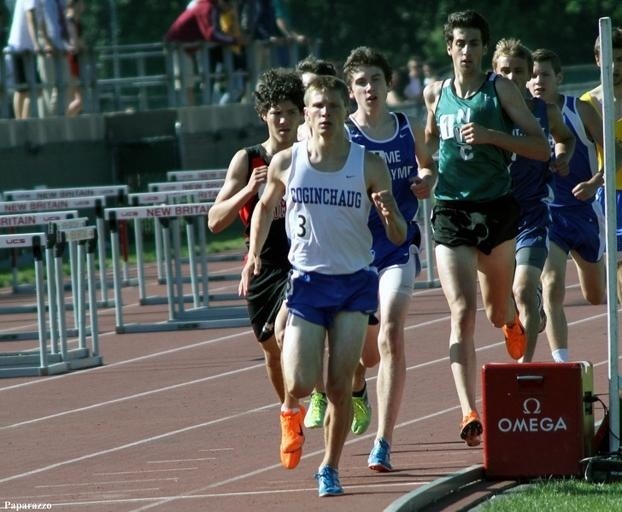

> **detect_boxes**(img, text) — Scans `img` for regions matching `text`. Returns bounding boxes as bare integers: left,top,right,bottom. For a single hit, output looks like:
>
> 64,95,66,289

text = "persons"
164,0,247,105
208,0,242,103
272,0,306,68
525,47,607,362
296,52,379,435
491,37,576,363
36,1,78,118
66,1,84,117
385,68,410,106
579,27,622,305
403,55,424,99
423,8,551,447
342,46,438,473
422,62,438,87
237,74,408,498
8,1,53,119
207,67,304,470
249,0,286,77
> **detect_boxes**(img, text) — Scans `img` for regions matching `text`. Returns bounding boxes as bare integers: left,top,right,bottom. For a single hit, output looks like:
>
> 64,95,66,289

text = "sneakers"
279,405,307,452
459,411,483,447
304,393,328,428
537,286,547,333
314,464,344,498
368,437,392,472
503,312,527,360
351,390,371,435
280,447,302,469
